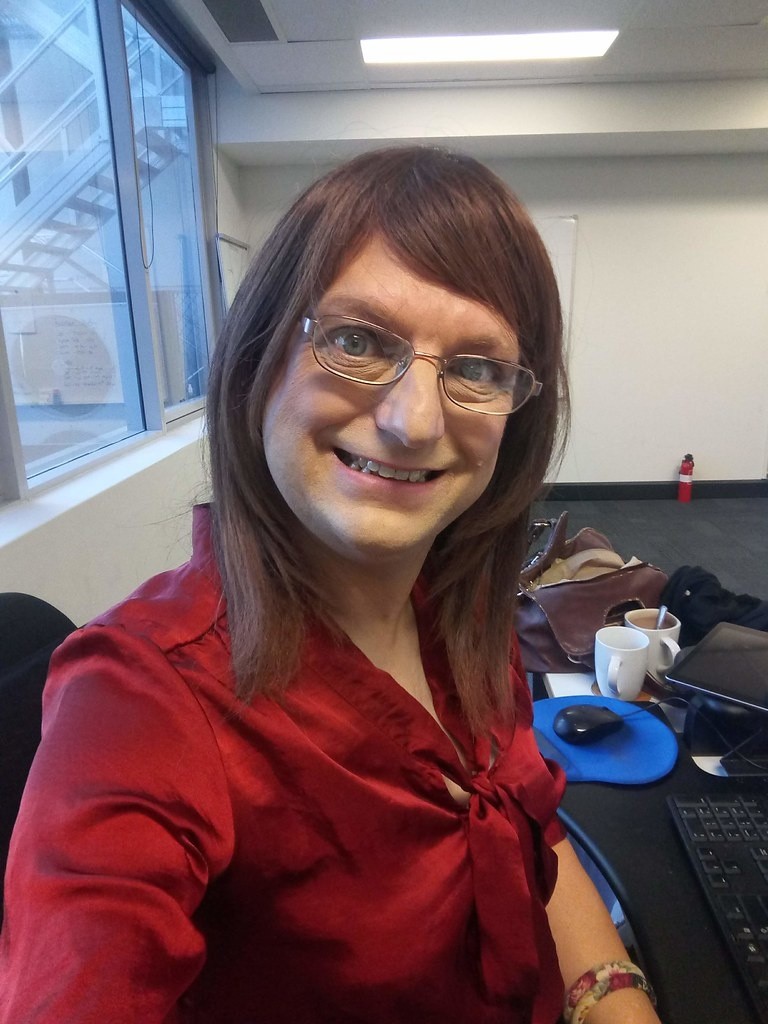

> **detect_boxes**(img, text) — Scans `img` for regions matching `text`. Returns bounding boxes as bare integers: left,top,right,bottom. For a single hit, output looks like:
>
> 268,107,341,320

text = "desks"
528,672,767,1024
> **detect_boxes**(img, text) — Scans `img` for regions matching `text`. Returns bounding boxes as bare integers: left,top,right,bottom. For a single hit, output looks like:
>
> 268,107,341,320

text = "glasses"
299,314,544,417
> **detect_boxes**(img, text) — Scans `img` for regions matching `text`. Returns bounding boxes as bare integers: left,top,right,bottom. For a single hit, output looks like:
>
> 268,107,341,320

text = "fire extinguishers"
678,453,695,503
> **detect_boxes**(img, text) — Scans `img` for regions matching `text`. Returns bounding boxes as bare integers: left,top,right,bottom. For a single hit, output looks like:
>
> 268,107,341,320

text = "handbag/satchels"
514,509,671,673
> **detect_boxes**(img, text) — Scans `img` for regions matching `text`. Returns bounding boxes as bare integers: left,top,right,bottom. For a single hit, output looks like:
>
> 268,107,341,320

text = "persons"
0,147,661,1024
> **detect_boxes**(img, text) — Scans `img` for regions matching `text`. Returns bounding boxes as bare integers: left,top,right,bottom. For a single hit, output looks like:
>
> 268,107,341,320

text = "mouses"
553,705,624,743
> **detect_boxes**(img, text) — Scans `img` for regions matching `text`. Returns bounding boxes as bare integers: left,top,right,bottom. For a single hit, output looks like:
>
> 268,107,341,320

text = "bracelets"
570,972,658,1024
560,960,646,1024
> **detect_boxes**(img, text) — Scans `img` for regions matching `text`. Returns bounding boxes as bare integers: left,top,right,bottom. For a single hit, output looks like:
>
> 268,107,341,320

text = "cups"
624,608,682,683
595,626,650,702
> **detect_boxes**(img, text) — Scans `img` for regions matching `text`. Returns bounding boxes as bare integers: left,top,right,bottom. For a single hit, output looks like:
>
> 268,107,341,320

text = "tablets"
664,622,768,716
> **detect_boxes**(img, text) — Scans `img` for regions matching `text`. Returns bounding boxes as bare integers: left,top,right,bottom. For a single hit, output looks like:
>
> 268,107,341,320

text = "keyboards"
666,790,767,1024
